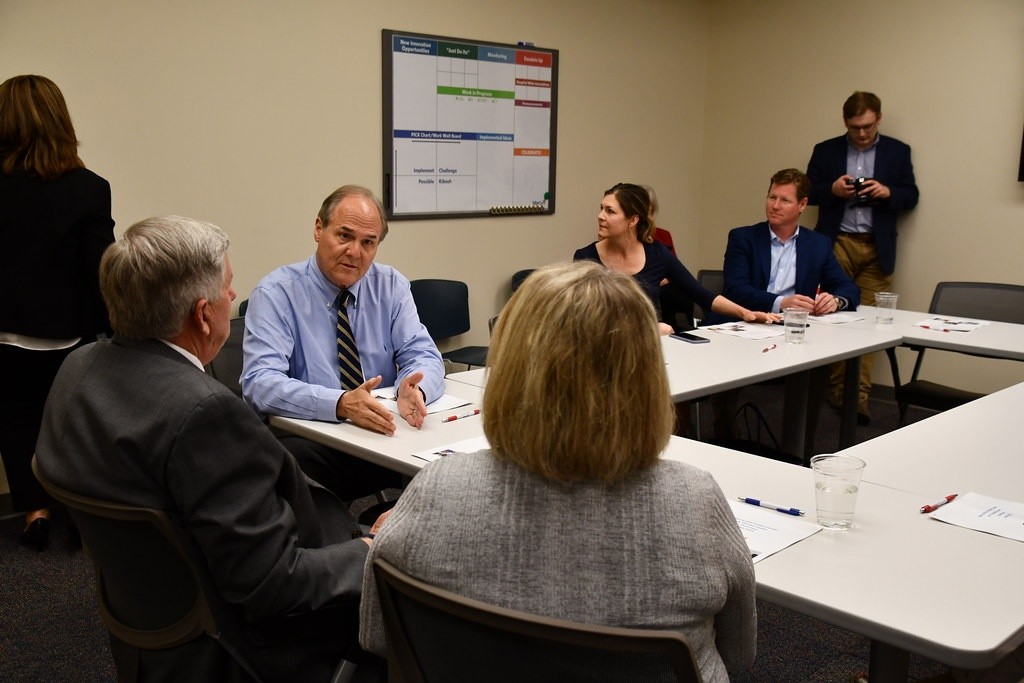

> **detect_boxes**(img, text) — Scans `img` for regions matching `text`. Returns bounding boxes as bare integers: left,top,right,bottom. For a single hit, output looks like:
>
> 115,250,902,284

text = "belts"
839,231,872,241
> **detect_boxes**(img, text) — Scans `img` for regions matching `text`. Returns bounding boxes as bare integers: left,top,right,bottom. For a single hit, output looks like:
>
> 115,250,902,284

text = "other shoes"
831,398,873,425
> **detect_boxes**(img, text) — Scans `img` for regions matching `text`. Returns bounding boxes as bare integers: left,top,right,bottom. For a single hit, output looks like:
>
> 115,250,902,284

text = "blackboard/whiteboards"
382,28,560,220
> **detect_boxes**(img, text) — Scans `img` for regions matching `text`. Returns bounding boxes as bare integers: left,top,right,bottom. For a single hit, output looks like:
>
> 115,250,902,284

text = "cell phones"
668,333,710,343
772,320,810,328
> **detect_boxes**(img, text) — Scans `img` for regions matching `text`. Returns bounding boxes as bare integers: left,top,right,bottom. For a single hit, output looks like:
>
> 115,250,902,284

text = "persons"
807,92,920,427
1,68,114,553
710,166,860,465
572,185,786,330
242,184,450,507
358,255,760,683
38,215,364,683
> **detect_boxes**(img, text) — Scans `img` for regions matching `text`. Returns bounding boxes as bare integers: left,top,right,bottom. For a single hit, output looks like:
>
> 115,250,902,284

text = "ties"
335,290,364,391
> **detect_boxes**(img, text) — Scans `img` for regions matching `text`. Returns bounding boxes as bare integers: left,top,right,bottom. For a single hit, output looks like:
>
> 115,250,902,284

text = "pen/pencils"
738,496,805,517
815,284,821,300
443,409,482,423
921,493,958,514
919,325,949,332
763,344,777,353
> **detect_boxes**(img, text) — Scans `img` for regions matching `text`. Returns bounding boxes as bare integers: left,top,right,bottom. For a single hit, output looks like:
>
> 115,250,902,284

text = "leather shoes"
23,508,51,545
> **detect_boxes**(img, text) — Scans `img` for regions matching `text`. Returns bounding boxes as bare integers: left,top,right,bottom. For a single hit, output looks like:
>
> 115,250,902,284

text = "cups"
810,454,866,529
782,307,810,344
875,292,900,324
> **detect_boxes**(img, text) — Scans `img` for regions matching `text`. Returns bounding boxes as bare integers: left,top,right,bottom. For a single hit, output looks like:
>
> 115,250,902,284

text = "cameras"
846,177,876,207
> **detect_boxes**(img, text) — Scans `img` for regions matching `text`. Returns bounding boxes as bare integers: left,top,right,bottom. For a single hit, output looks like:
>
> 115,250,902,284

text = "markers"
518,41,535,45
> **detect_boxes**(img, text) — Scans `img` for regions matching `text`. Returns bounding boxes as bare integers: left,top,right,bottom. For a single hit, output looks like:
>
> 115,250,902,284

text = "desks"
813,381,1024,502
446,320,908,465
269,379,1024,683
810,303,1024,362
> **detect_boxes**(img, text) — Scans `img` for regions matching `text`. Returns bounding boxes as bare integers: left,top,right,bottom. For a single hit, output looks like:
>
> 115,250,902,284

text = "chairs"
865,281,1024,423
369,554,703,683
202,317,244,399
30,455,364,683
402,267,537,373
